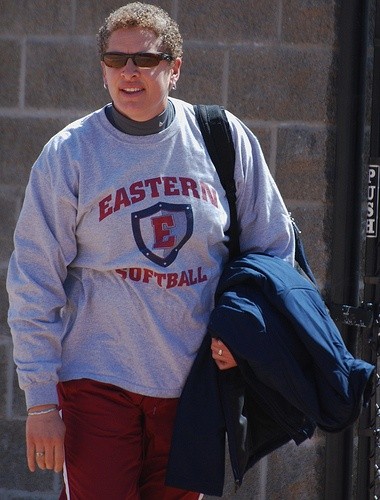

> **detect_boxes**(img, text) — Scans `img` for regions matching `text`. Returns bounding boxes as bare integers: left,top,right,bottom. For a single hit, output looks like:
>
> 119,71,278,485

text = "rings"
35,452,44,456
218,349,222,356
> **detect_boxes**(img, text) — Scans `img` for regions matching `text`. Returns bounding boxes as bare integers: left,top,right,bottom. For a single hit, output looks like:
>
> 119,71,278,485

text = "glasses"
102,52,173,68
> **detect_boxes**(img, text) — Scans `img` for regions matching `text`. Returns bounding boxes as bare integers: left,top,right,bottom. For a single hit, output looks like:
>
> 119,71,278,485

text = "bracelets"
27,407,58,416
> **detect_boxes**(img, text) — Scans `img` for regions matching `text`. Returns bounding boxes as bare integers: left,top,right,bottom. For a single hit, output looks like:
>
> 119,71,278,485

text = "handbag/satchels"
194,104,319,297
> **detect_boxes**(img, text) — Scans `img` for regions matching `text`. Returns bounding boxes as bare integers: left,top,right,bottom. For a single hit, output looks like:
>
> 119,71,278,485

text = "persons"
5,1,294,500
